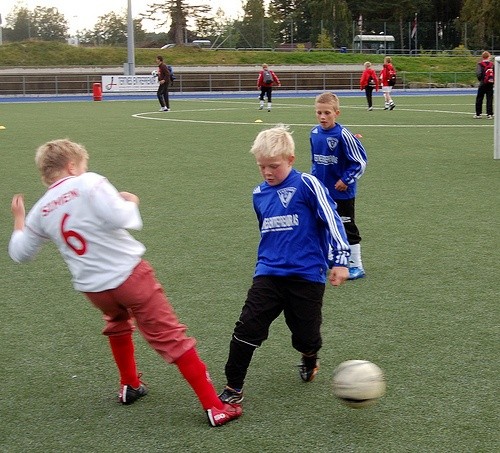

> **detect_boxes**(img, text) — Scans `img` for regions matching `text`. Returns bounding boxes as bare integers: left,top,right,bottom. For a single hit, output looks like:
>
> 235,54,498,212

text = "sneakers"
218,386,244,404
300,352,319,383
119,373,146,405
346,266,366,280
205,402,243,426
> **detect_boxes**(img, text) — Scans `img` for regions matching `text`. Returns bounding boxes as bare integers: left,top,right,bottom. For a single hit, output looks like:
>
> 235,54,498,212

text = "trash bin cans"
341,47,347,53
93,83,102,100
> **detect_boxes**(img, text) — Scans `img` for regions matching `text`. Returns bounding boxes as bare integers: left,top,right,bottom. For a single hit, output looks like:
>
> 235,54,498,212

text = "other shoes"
367,107,372,111
159,107,171,111
384,107,389,110
389,104,395,111
473,114,483,119
487,114,493,119
260,106,263,110
268,108,271,113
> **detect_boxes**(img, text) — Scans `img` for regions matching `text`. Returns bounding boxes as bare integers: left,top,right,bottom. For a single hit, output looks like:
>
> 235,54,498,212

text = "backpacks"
480,61,494,82
264,70,273,84
367,72,376,88
384,66,396,86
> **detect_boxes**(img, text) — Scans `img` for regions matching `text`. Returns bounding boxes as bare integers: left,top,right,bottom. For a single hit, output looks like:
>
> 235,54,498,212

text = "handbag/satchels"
166,65,175,80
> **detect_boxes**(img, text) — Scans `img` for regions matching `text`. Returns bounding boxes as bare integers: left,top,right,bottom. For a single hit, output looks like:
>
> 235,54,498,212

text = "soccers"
152,70,158,77
330,360,384,408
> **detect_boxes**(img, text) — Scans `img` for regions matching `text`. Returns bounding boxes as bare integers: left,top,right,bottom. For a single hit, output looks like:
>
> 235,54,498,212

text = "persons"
359,61,379,111
379,56,395,110
256,63,281,112
473,50,494,119
308,91,367,280
164,63,176,87
155,56,171,111
9,138,244,427
216,123,352,403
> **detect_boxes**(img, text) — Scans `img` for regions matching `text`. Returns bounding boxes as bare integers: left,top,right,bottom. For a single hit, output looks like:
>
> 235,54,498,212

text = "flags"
410,19,417,38
